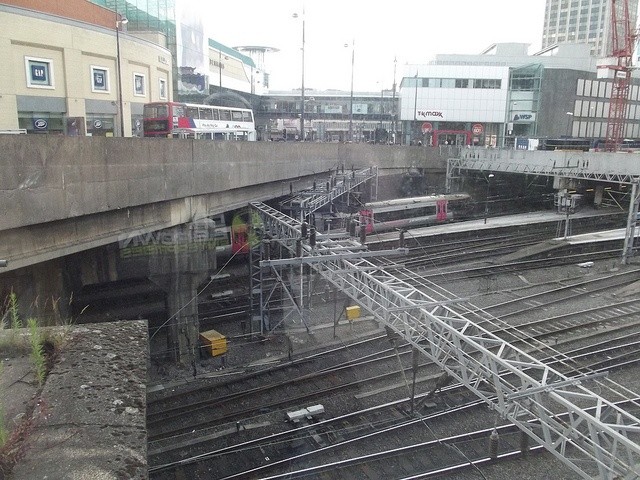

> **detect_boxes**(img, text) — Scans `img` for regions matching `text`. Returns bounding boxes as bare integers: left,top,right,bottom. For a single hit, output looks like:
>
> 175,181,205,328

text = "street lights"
344,38,355,136
116,18,130,138
292,12,305,142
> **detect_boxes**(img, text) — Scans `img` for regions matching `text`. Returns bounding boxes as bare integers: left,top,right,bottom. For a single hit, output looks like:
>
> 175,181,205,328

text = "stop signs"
472,124,483,136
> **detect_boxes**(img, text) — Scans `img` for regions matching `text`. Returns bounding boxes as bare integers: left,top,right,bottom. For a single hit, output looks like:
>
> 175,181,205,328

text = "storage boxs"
199,328,227,357
346,306,361,321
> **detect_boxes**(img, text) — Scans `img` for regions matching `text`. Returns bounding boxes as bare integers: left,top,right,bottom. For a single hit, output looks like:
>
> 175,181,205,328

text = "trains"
359,193,476,235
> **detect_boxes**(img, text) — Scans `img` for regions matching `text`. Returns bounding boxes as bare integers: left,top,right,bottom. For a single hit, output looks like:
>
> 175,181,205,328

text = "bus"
144,102,258,143
